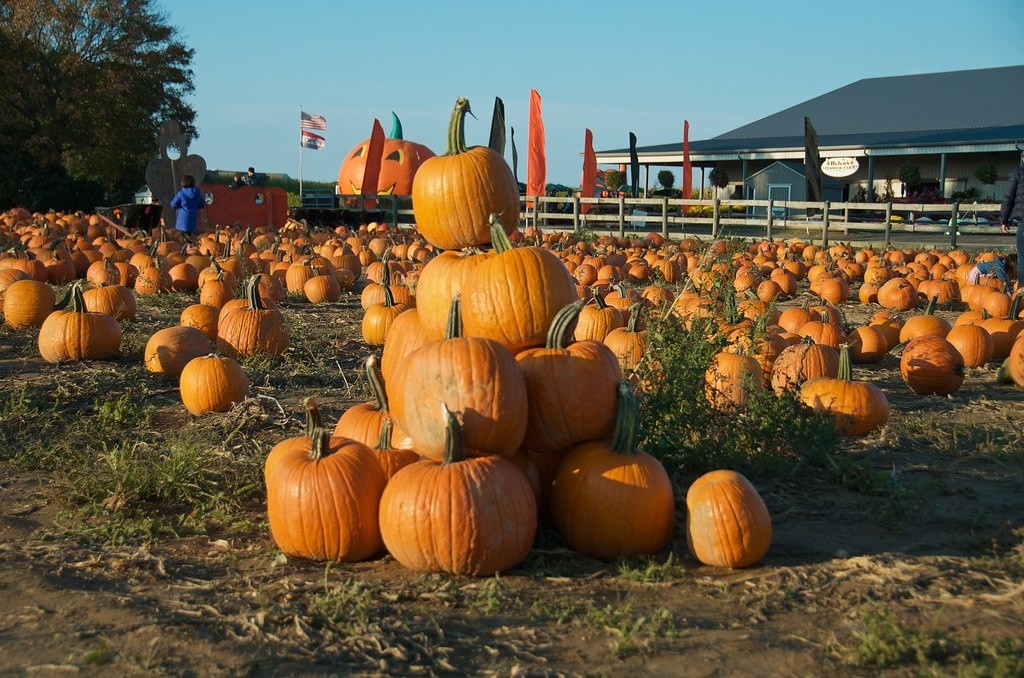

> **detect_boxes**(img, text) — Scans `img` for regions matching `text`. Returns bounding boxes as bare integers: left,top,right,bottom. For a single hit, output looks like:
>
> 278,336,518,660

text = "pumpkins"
0,97,1024,577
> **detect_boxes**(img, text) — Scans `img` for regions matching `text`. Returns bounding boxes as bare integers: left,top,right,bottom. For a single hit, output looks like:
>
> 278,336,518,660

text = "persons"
169,175,204,244
241,167,263,187
231,173,246,192
966,253,1018,284
999,151,1024,287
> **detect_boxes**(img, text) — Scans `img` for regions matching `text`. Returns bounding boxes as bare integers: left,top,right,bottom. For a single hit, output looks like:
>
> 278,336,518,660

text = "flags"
301,130,326,151
300,111,326,131
